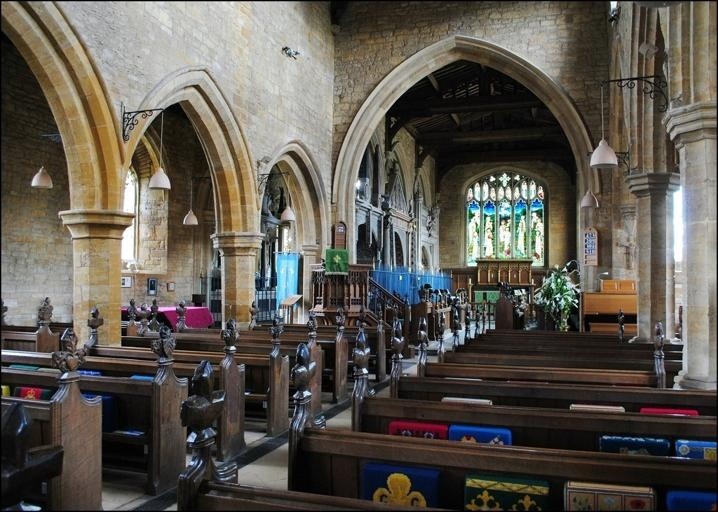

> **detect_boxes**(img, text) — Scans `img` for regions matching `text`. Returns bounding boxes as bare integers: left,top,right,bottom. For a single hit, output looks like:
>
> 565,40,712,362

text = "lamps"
120,103,172,194
578,150,631,209
27,128,70,194
182,171,215,229
586,71,669,170
255,168,299,226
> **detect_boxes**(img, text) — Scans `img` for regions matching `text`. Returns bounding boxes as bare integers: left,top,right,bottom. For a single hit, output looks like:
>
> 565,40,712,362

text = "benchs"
0,279,717,512
